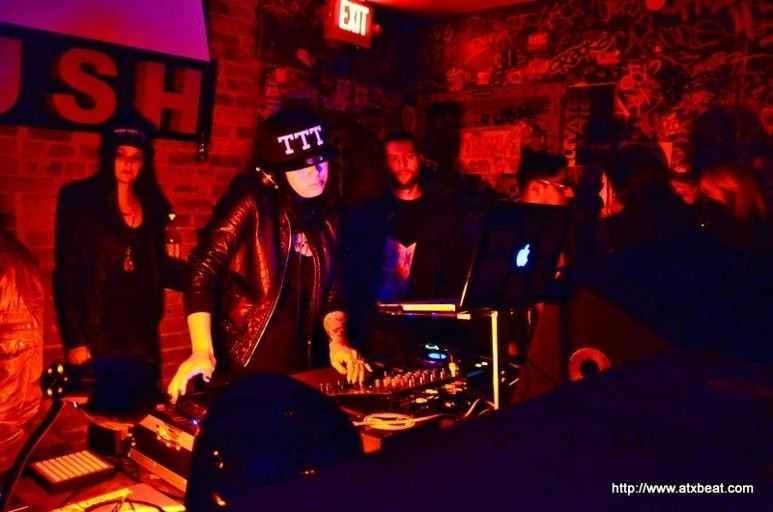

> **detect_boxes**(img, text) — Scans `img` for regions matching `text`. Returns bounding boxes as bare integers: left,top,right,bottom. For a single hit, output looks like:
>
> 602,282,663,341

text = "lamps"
0,355,162,512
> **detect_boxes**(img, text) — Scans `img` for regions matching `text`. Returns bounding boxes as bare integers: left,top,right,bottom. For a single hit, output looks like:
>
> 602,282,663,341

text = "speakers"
509,287,673,406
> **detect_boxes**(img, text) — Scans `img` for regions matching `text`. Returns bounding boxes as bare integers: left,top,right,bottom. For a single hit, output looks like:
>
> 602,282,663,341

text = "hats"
103,128,153,164
257,109,340,172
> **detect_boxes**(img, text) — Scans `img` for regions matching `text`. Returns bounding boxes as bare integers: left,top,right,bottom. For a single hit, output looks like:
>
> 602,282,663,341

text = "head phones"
255,164,282,192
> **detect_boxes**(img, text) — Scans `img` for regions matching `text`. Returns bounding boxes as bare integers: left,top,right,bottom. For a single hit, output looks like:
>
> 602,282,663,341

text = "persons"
53,121,189,452
340,129,458,355
163,103,372,403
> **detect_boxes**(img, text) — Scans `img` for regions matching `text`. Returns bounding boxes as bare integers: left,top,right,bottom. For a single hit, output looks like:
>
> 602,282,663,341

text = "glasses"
539,178,569,189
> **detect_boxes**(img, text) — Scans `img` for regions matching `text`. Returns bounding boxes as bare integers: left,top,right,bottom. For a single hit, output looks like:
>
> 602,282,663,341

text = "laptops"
378,202,573,312
